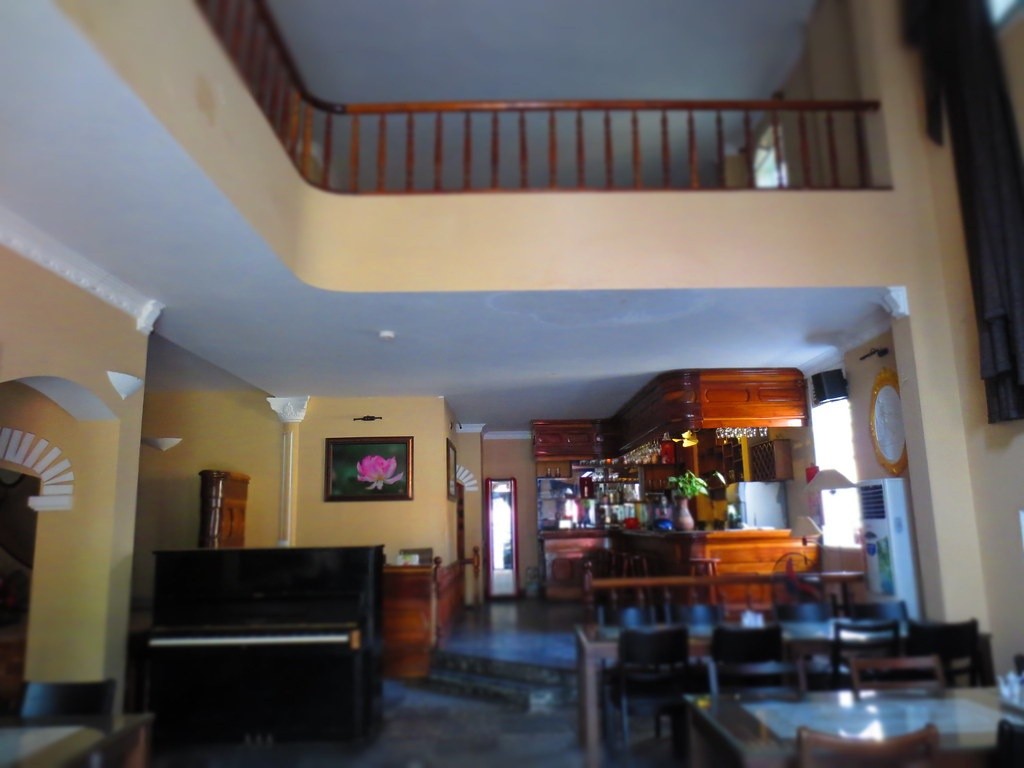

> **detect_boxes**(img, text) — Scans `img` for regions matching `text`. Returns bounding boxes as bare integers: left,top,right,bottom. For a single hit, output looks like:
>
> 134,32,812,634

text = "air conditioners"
857,476,923,624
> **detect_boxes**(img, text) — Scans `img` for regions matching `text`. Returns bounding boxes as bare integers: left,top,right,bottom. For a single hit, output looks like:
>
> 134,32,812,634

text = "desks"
684,684,1024,768
574,616,909,768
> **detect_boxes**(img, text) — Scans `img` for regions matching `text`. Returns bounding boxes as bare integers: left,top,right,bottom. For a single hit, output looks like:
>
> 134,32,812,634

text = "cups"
715,427,767,439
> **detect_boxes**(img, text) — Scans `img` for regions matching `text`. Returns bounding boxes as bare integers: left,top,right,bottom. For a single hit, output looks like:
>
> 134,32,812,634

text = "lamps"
811,368,851,405
801,467,860,496
579,431,676,465
680,430,698,447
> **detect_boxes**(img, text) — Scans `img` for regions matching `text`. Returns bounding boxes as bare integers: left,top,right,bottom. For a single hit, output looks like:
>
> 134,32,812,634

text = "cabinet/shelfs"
196,469,252,550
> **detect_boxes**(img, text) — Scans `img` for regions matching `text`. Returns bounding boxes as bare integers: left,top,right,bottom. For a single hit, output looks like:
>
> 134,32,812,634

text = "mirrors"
870,366,910,478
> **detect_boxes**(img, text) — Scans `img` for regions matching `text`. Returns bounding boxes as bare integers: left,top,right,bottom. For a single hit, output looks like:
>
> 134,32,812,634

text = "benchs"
591,570,867,618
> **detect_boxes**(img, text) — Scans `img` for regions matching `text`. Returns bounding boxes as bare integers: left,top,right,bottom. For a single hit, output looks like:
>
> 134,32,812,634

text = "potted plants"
669,471,709,530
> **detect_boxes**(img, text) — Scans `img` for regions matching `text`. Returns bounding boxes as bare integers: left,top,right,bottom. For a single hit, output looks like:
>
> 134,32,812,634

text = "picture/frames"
323,435,415,502
446,437,456,502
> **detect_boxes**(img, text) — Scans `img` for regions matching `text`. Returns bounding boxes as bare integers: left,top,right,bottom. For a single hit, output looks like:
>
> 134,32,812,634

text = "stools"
592,544,723,601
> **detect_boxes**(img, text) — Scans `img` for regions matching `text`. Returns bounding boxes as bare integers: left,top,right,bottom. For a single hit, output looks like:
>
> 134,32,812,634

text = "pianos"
144,543,389,752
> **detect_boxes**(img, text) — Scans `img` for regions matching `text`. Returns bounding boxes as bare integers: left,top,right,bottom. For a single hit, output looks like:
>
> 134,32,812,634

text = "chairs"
592,602,1024,768
